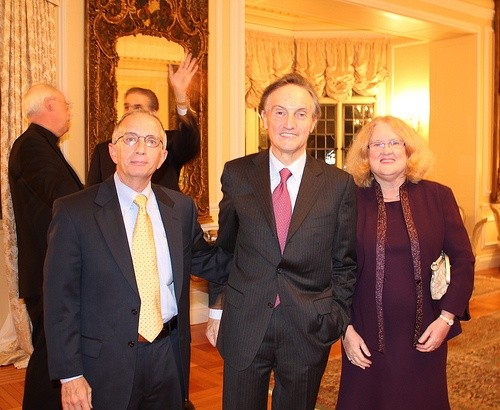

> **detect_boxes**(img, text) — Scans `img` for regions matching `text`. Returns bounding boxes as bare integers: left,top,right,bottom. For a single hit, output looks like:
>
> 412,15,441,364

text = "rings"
348,358,353,361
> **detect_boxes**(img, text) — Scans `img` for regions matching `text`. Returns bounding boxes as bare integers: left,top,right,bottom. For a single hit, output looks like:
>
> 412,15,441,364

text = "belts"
136,315,179,344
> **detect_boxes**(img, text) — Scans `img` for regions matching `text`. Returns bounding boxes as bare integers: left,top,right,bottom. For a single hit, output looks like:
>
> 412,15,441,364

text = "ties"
131,195,164,343
271,168,293,309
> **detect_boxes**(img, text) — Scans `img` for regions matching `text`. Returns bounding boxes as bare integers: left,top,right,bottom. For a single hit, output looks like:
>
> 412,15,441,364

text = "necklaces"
383,195,400,199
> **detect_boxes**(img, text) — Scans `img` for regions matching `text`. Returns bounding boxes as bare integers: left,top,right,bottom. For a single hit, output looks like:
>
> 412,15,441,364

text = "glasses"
114,132,163,150
47,97,70,111
368,137,405,152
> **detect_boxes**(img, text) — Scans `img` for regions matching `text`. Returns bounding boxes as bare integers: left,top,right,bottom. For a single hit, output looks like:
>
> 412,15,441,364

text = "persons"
206,73,357,410
7,83,85,410
84,52,201,191
336,115,475,410
44,110,218,410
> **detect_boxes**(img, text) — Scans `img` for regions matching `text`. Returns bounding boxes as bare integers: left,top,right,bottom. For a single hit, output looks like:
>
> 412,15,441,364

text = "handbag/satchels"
430,250,451,300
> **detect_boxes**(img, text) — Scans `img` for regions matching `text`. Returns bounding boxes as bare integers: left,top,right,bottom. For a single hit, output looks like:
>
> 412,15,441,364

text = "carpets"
269,310,500,410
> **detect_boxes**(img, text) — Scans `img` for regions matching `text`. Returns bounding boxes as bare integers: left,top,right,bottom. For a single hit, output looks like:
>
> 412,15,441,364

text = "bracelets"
174,99,188,105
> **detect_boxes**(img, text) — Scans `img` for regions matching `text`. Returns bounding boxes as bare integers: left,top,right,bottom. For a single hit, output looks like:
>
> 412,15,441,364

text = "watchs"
440,315,454,325
